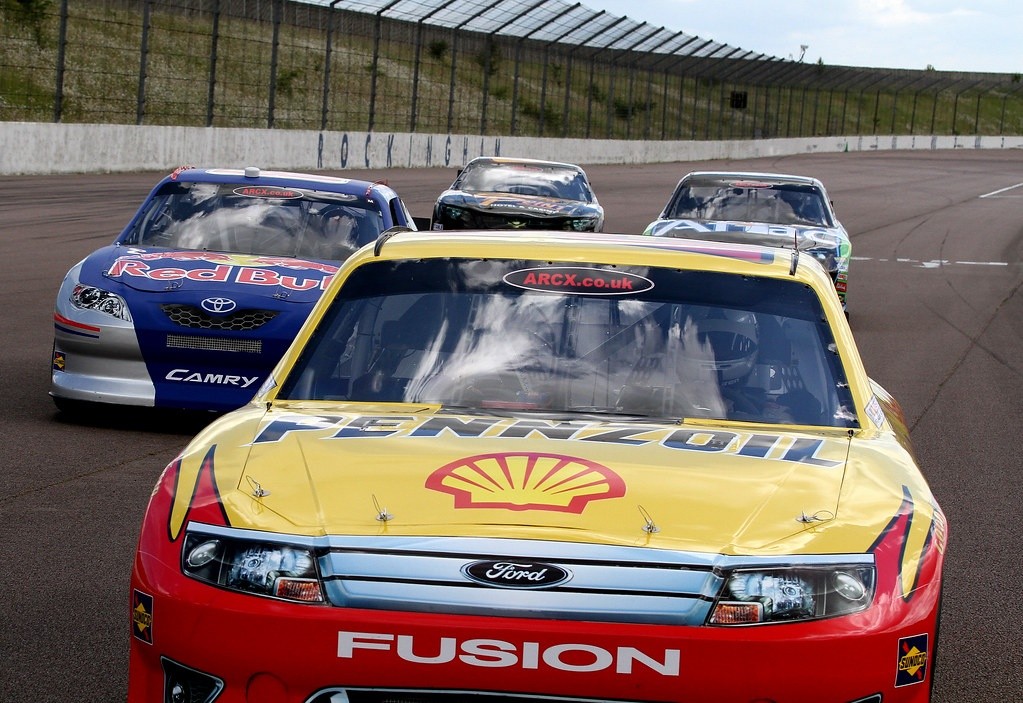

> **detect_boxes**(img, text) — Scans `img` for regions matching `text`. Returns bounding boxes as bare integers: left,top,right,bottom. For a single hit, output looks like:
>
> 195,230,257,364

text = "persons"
633,303,812,429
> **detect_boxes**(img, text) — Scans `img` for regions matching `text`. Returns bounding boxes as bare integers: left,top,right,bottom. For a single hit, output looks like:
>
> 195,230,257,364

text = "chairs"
358,291,493,403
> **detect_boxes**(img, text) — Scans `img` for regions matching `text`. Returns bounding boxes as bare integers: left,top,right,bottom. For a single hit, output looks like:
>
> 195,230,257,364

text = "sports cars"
127,224,953,703
643,171,853,321
429,157,606,237
49,165,420,418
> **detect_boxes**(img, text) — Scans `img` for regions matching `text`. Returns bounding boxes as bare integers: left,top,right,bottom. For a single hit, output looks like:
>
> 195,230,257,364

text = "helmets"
777,189,804,216
673,305,758,384
319,206,361,248
